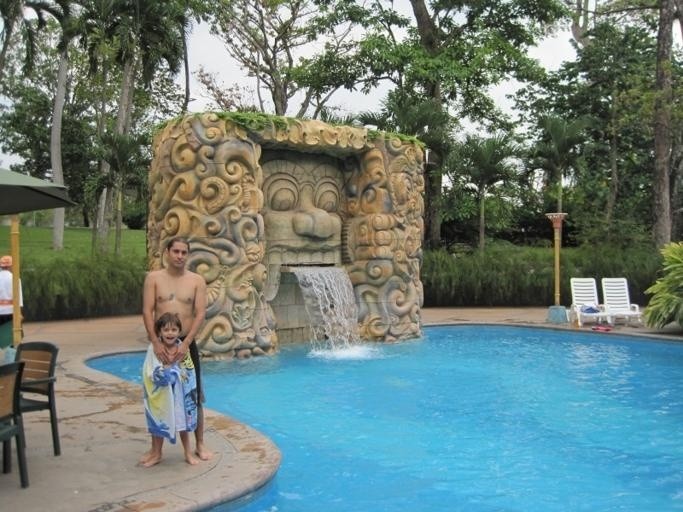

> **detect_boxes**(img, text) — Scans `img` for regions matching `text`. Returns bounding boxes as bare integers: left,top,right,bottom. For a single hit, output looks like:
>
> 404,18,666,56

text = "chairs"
14,341,61,456
566,277,610,327
601,277,644,328
0,360,30,488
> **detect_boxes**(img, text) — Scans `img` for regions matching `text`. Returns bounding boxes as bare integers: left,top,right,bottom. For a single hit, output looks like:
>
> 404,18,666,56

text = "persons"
140,314,200,468
1,255,25,351
138,240,213,463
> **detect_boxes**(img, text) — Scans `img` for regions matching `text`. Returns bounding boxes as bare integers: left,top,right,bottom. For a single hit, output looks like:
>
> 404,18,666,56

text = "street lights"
544,213,569,324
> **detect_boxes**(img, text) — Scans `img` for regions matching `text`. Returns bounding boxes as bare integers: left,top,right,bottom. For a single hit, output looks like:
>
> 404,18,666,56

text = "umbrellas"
0,166,79,216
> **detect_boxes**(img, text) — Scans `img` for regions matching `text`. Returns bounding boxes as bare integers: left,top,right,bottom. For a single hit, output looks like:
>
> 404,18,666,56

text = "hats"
0,254,13,268
